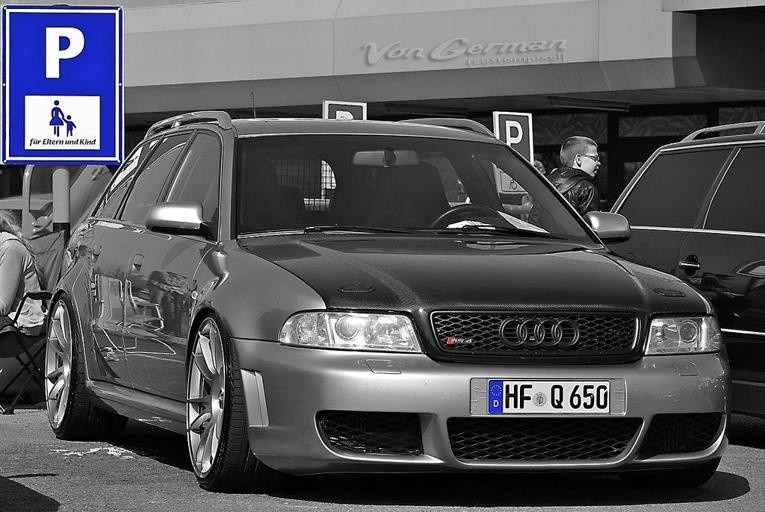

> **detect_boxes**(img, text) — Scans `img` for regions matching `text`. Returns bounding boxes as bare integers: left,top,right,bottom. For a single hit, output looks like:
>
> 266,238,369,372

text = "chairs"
1,291,57,415
238,161,451,231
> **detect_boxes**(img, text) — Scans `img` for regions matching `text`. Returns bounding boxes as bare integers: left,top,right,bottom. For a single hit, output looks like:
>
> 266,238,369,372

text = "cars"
40,91,734,496
595,118,765,426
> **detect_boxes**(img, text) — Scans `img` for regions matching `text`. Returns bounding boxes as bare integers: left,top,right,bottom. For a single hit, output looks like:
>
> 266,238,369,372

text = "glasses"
581,154,599,162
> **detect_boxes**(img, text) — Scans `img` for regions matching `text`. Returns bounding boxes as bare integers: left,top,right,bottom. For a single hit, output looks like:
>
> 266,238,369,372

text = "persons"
0,211,45,357
527,133,603,233
